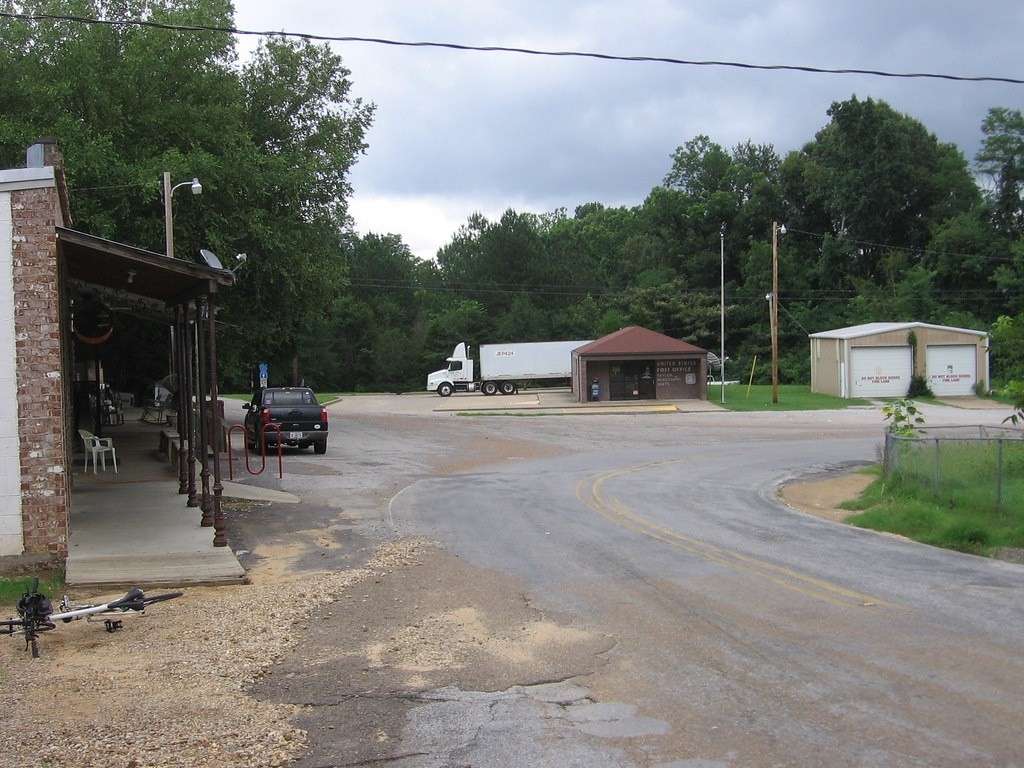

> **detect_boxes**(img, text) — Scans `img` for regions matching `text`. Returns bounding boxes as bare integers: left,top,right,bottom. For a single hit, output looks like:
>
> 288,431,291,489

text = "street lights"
772,222,786,404
169,176,203,360
719,222,730,402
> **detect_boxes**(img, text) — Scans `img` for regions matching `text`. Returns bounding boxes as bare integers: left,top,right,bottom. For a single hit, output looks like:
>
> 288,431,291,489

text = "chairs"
98,388,125,426
134,389,173,426
78,429,118,475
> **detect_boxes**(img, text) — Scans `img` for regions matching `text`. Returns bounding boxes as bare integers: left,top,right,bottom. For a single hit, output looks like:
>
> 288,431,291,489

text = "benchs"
172,439,197,481
160,429,180,463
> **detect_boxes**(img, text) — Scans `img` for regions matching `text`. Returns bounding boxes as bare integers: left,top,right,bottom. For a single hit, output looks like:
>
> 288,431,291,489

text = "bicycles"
1,576,185,658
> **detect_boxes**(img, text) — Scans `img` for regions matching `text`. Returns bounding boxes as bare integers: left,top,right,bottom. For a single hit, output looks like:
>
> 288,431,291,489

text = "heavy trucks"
426,340,596,398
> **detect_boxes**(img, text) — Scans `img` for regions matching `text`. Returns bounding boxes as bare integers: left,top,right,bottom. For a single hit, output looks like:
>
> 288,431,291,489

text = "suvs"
242,385,329,454
